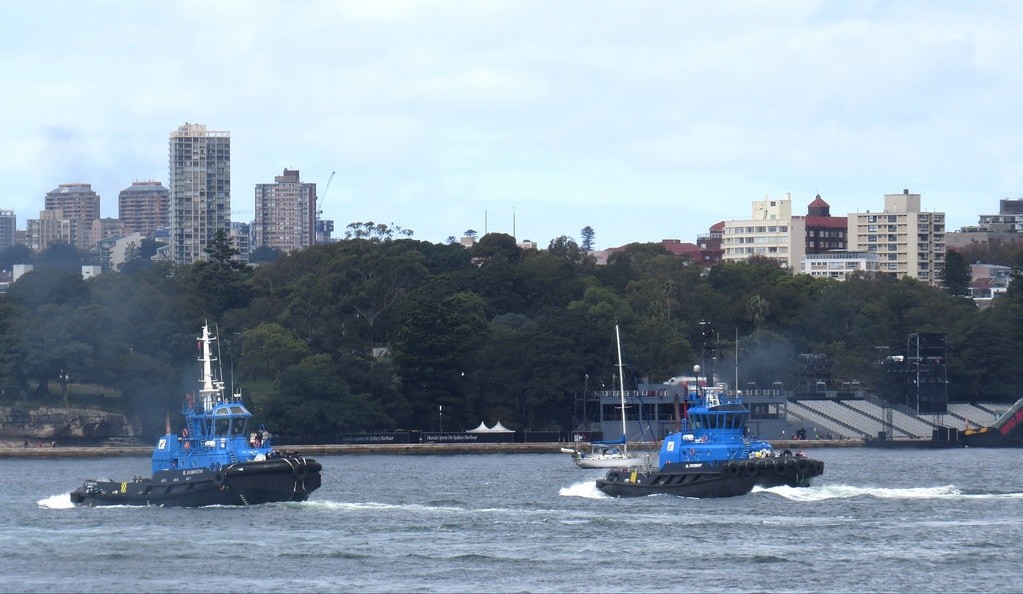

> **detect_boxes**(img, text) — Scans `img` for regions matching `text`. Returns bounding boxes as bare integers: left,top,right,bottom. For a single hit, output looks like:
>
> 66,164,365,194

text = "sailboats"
559,322,651,470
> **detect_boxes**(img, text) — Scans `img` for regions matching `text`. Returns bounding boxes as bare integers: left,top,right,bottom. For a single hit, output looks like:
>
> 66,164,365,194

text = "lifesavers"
183,428,188,436
253,440,260,447
293,463,308,480
795,460,809,474
814,463,822,474
212,471,229,487
721,461,760,476
775,464,788,476
690,448,695,455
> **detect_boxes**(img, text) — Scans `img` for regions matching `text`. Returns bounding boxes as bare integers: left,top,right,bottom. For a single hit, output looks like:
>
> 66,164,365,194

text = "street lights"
692,364,701,397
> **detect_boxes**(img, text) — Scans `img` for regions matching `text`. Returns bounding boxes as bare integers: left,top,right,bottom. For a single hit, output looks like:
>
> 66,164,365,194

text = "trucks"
663,375,719,396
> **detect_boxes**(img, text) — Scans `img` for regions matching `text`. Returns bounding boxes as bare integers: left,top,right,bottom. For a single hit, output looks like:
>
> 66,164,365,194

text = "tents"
466,421,516,433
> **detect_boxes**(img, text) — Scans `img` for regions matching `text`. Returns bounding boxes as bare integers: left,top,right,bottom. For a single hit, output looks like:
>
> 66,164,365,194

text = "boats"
595,319,824,498
70,316,323,508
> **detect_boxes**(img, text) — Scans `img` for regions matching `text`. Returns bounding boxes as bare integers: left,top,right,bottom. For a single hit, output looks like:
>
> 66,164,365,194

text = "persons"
25,440,56,447
749,432,847,459
